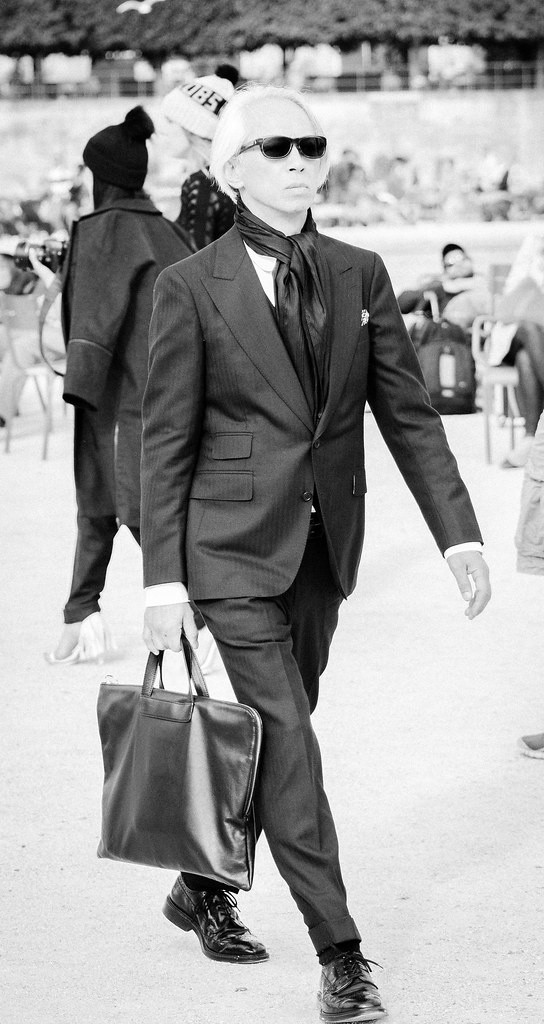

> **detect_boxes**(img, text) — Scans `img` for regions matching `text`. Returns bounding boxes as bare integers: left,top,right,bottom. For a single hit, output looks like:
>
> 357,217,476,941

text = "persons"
0,199,541,425
160,75,242,248
502,400,544,756
41,98,201,663
130,88,492,1023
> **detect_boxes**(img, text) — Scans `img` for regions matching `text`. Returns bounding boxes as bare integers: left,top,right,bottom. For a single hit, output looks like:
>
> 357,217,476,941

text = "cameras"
13,239,68,273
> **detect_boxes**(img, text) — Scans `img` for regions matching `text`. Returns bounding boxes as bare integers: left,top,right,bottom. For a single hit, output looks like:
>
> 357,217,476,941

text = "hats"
82,105,155,189
160,64,239,141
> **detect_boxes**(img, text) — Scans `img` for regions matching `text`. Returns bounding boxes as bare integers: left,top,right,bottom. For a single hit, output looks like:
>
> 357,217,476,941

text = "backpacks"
411,321,480,417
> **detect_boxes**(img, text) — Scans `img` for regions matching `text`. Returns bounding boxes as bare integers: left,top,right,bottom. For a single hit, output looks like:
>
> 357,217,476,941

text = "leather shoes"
161,876,270,964
317,952,387,1023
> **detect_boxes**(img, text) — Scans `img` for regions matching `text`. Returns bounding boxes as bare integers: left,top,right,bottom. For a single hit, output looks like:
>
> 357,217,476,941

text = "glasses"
233,135,327,159
78,162,88,177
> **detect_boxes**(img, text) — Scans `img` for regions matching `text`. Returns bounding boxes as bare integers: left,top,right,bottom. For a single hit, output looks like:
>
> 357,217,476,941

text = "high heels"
198,639,218,675
47,612,109,663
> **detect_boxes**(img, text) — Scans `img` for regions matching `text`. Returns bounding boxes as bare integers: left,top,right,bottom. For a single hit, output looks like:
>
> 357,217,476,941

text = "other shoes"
518,733,544,761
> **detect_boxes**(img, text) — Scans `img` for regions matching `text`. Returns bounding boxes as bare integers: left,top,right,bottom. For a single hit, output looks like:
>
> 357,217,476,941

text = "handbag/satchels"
97,629,265,893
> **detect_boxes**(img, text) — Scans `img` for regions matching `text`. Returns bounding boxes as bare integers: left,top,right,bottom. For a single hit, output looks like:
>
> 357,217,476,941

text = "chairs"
472,315,521,466
0,290,69,462
